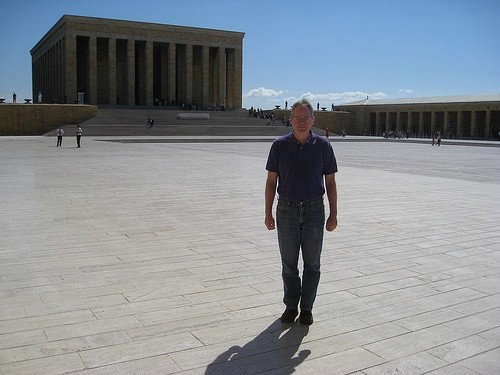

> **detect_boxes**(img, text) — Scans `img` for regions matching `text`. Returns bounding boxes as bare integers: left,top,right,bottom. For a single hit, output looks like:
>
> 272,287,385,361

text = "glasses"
291,115,313,122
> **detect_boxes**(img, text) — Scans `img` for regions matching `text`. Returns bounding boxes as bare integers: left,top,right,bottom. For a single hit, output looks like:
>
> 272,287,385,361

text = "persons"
12,92,17,104
317,102,320,110
75,125,83,150
332,102,334,111
56,126,64,148
342,128,347,138
326,127,329,138
432,125,458,146
265,99,339,326
155,97,225,112
37,91,42,103
382,127,414,140
146,116,155,128
248,100,293,127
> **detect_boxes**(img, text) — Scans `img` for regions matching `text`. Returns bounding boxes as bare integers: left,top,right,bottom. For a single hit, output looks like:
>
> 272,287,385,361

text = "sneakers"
300,311,313,325
280,309,298,323
